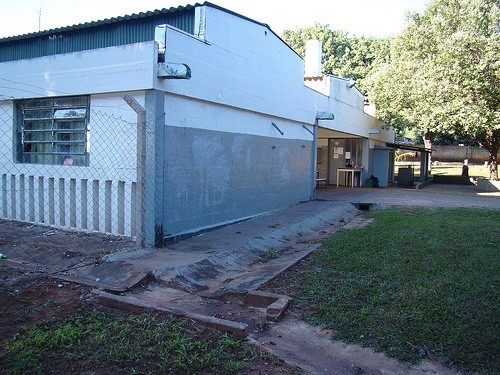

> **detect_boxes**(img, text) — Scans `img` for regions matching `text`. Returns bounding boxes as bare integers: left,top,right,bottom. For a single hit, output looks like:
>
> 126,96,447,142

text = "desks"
336,168,364,189
